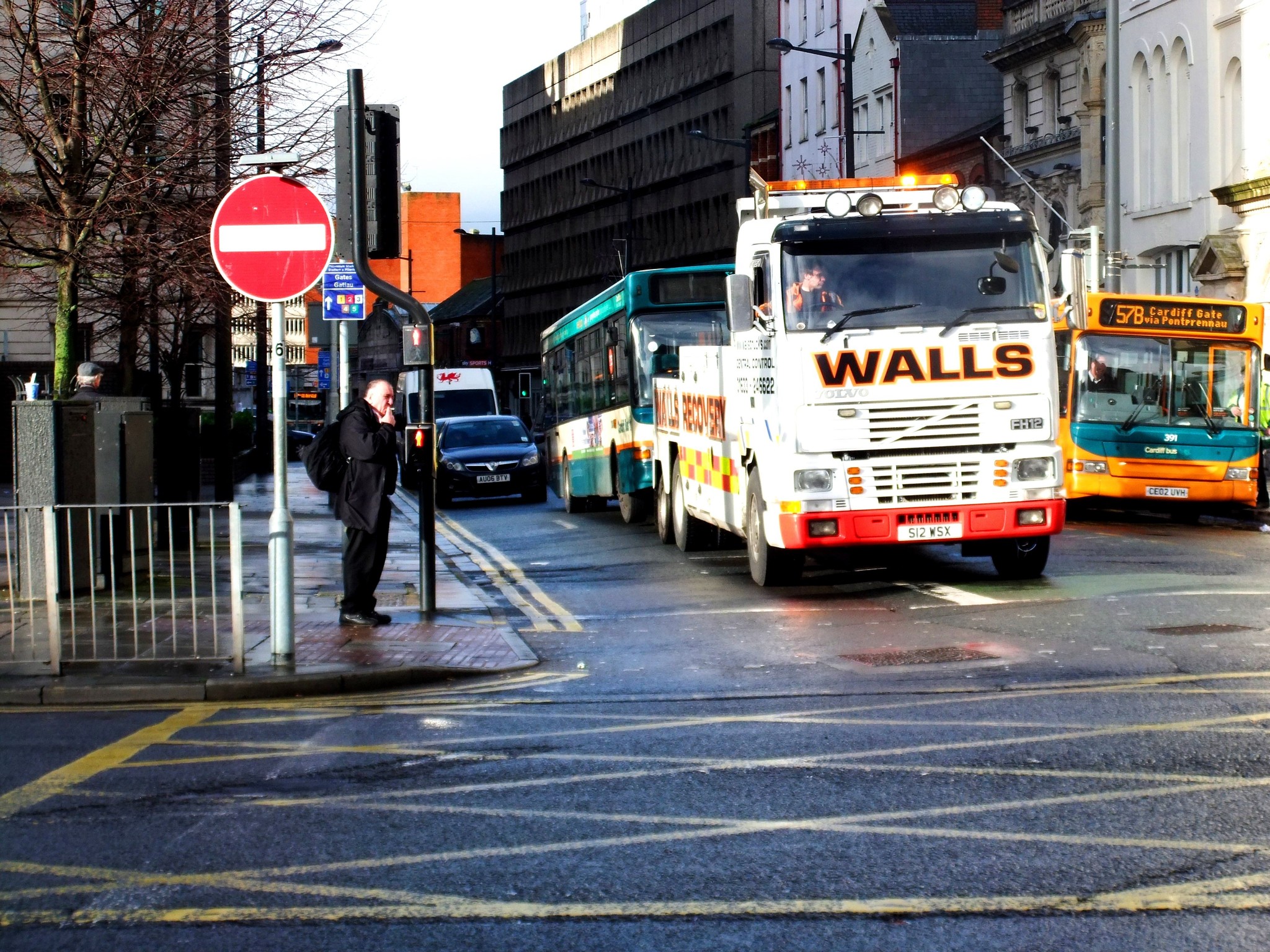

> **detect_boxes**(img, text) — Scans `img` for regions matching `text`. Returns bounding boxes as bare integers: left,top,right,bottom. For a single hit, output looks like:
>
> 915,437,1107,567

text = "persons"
756,258,844,319
68,363,104,399
326,380,396,627
1228,366,1270,507
1063,354,1117,410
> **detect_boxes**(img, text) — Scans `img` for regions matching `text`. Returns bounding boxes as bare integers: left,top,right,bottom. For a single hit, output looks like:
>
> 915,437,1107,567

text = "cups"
25,383,39,401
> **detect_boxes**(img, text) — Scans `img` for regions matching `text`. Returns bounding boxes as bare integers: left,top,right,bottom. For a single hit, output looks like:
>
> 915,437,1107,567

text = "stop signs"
209,172,336,304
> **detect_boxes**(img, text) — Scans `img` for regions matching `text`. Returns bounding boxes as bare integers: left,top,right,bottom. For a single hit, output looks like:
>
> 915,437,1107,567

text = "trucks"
652,174,1087,591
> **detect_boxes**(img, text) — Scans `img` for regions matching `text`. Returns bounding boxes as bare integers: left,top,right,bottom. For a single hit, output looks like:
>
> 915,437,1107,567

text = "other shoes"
1251,501,1270,510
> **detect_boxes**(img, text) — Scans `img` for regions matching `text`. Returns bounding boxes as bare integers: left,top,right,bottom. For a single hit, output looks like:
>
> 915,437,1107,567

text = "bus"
1049,290,1270,518
539,262,735,526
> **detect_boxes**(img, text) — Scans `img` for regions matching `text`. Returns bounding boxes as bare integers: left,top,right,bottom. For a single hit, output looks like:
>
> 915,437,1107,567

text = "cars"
251,417,317,462
436,414,546,511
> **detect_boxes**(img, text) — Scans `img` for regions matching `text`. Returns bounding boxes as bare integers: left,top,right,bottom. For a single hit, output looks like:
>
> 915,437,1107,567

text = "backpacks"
302,410,371,494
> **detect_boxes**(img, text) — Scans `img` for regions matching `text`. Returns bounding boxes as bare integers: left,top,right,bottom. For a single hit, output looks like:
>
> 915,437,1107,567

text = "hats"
77,362,105,377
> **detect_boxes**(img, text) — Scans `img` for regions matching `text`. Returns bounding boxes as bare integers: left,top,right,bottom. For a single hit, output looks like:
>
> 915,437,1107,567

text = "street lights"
253,32,344,477
763,33,855,212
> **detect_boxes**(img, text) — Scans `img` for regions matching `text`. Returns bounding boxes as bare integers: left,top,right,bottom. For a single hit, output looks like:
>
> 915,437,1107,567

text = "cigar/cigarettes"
390,408,395,410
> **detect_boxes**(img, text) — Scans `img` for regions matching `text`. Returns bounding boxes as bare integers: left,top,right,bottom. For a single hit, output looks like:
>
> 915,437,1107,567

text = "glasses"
97,373,104,377
812,272,826,278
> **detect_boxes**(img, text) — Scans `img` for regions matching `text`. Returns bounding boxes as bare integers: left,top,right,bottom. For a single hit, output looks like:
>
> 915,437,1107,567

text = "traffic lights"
518,373,531,398
401,324,435,366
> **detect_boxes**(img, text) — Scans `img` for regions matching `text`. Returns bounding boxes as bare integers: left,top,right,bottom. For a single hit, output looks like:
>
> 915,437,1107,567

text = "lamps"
1053,163,1072,170
990,180,1010,188
997,135,1011,143
1058,116,1071,124
1021,168,1040,180
1025,126,1038,134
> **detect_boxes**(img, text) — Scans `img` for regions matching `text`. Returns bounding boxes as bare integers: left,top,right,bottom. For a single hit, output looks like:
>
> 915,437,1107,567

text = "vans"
394,368,500,490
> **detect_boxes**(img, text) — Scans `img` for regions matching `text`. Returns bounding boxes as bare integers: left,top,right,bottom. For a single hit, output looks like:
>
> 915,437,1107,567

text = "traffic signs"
322,263,365,321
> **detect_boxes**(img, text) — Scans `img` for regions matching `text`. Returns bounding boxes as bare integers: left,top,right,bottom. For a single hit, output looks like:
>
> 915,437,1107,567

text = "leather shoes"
338,608,377,627
361,610,392,625
